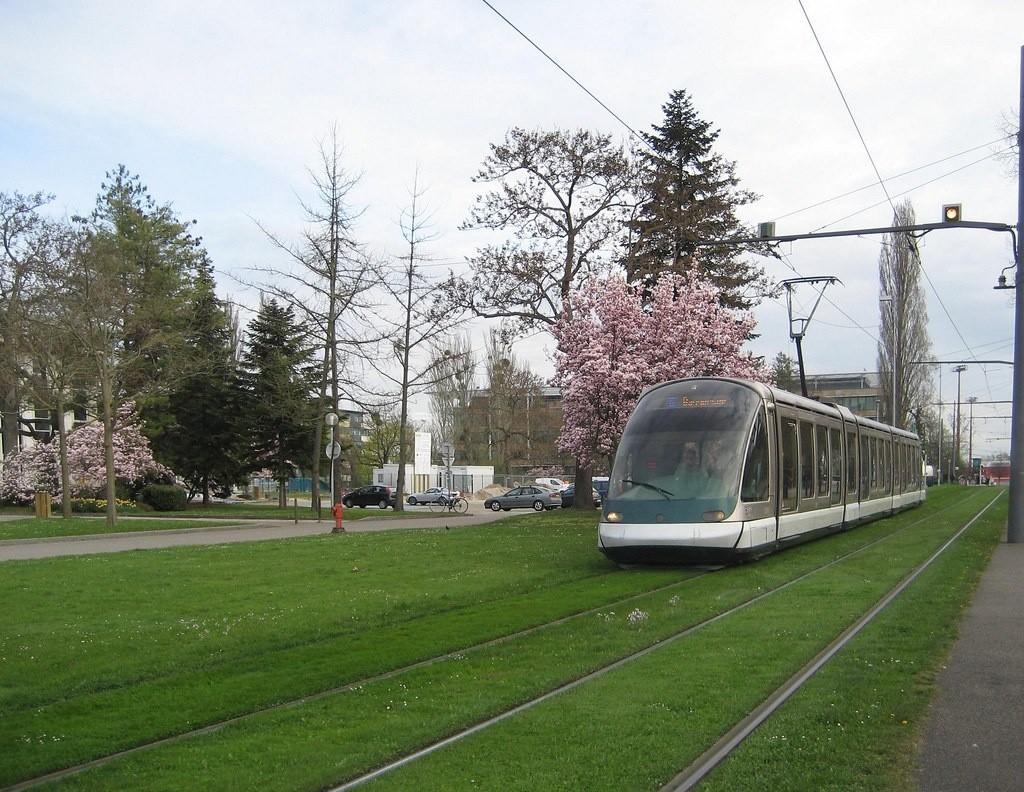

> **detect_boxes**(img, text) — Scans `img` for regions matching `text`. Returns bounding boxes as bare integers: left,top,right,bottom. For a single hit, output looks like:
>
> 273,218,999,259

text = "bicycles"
429,488,468,514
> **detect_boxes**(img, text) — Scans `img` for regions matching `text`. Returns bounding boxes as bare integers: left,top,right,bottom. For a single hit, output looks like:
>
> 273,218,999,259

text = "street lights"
966,396,977,480
949,364,967,479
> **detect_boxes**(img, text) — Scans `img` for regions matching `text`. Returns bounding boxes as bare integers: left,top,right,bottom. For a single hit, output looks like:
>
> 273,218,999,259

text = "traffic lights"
942,203,962,224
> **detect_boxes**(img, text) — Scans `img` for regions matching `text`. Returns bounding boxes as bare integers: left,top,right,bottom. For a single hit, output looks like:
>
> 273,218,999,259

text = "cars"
405,487,460,507
483,485,562,511
341,484,396,510
534,476,609,508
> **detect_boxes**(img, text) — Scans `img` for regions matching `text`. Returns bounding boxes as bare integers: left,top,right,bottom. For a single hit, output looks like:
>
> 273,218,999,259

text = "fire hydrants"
331,503,344,533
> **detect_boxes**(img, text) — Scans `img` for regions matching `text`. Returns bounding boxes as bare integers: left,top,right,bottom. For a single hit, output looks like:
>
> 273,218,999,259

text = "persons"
673,447,710,497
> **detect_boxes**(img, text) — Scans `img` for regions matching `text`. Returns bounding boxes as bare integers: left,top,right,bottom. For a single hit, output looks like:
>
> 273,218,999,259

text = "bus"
599,377,929,570
